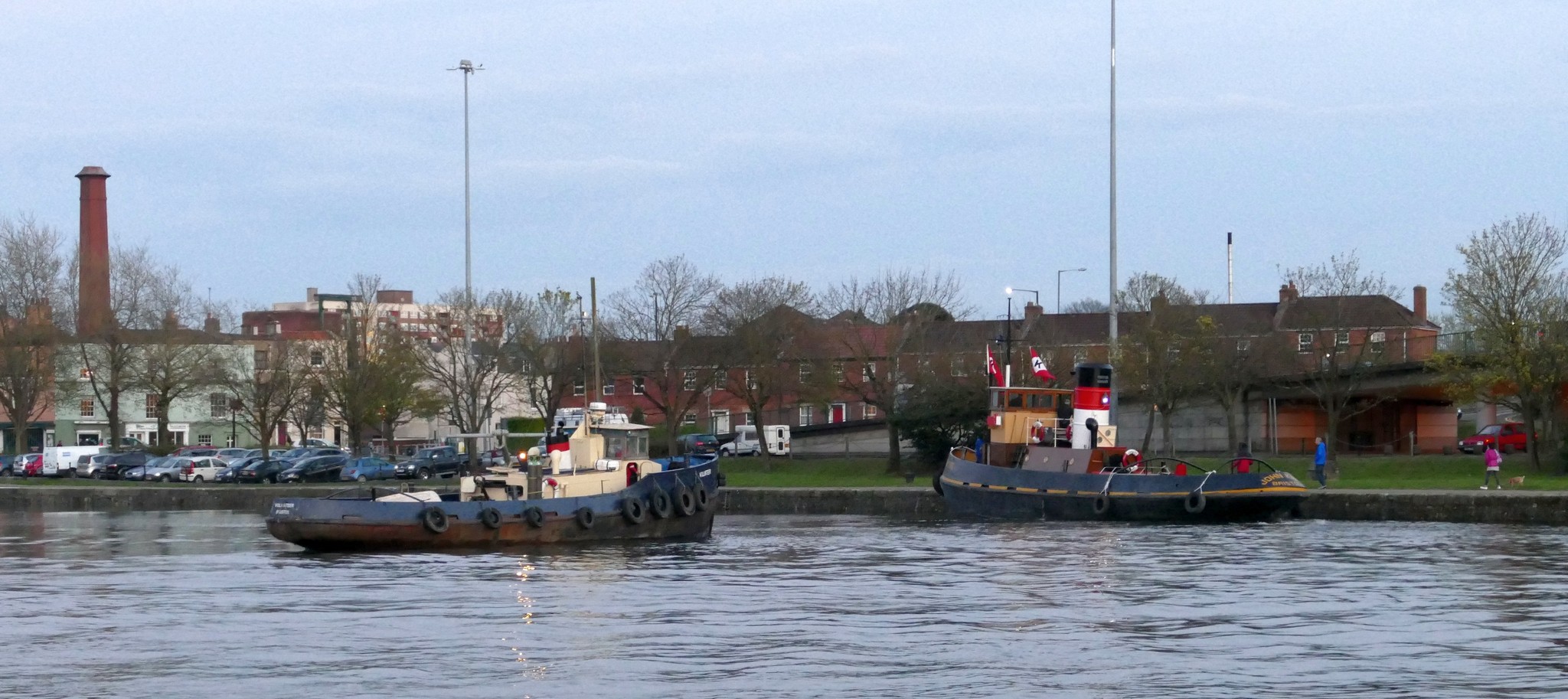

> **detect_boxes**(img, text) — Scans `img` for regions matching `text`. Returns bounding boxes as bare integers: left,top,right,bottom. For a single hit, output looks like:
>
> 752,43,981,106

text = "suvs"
675,434,720,455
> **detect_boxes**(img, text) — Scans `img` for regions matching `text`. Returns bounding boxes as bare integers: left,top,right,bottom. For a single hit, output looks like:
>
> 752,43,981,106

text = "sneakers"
1480,485,1488,489
1497,485,1501,489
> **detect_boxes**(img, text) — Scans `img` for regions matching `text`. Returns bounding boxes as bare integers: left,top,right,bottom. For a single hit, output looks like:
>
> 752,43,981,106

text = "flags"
1029,345,1057,383
987,344,1010,395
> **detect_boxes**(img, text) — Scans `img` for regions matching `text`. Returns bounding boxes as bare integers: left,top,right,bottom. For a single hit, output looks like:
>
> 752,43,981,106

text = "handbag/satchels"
1496,457,1502,464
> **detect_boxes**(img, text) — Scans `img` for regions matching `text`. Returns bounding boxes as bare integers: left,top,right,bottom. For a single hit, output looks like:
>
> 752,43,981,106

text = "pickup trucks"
394,445,483,481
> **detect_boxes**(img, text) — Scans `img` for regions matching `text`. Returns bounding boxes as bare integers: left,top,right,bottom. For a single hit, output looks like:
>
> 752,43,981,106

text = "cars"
1457,421,1538,453
77,436,394,485
479,448,519,471
0,453,43,477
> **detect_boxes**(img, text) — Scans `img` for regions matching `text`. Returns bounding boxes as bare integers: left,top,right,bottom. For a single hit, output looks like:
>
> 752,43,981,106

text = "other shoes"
1317,484,1327,489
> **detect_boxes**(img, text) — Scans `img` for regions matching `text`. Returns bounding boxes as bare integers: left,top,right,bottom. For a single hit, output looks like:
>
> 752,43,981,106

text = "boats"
939,337,1312,521
266,411,723,542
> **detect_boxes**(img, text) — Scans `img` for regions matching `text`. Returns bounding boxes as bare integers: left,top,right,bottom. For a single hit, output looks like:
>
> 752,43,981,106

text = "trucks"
44,446,105,477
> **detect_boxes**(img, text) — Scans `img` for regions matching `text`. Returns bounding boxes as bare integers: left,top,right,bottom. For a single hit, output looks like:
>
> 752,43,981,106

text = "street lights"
1005,288,1039,306
577,291,589,409
1057,267,1087,313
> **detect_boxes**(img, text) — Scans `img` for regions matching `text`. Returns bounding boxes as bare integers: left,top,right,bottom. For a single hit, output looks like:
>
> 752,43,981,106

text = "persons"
57,440,62,447
1315,437,1327,488
1232,442,1254,474
975,434,985,463
1479,442,1501,490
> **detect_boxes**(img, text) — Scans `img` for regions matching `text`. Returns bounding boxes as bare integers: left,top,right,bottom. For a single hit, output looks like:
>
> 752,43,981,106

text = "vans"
719,424,790,457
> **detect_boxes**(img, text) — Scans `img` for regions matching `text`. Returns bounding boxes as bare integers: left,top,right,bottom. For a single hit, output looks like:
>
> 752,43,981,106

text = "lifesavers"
541,478,559,499
1031,421,1044,444
1122,449,1141,472
1066,423,1073,443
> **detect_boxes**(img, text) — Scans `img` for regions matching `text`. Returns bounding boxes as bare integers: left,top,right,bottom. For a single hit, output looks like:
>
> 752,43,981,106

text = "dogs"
1509,475,1525,487
